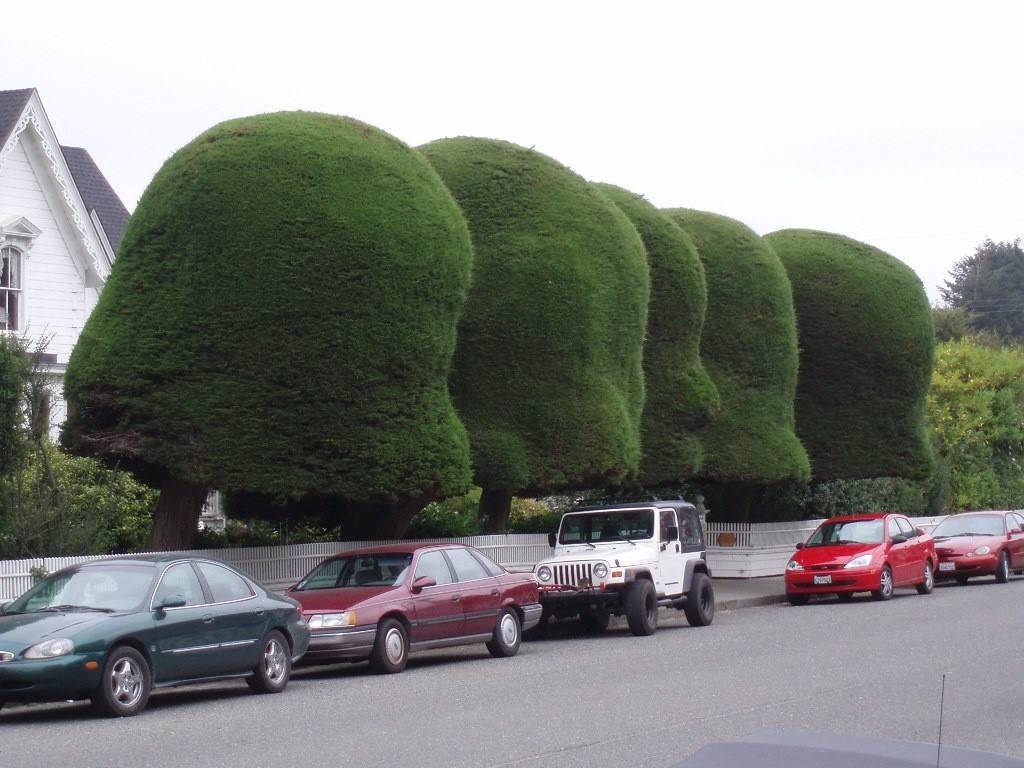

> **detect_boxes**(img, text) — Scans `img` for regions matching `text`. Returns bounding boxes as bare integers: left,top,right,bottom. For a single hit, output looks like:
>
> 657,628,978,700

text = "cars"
930,509,1024,585
281,541,543,674
784,513,939,606
1,555,311,717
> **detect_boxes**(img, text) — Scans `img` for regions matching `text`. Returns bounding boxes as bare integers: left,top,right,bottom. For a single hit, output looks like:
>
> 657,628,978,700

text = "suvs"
532,493,716,638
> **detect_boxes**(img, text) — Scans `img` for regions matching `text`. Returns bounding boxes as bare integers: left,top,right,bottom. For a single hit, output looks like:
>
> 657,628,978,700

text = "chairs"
600,521,619,540
354,557,378,585
